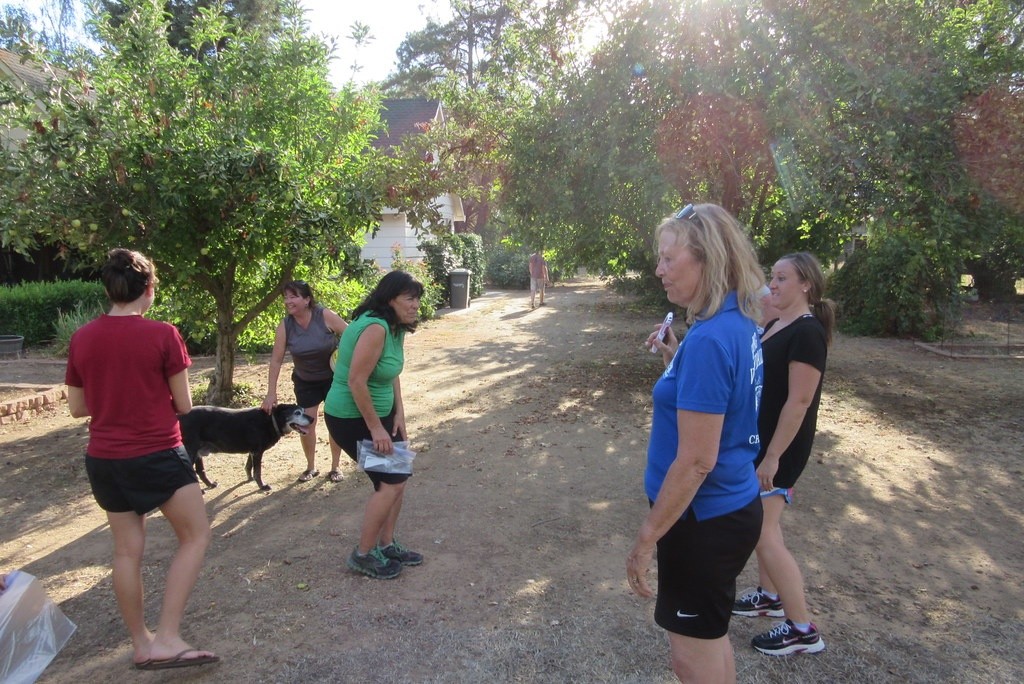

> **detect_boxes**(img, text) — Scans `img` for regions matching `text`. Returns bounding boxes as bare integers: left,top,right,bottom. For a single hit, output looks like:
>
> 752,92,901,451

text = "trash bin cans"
449,268,475,309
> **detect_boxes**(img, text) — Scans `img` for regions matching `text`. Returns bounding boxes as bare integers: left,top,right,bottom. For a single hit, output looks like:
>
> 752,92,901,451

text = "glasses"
675,203,704,233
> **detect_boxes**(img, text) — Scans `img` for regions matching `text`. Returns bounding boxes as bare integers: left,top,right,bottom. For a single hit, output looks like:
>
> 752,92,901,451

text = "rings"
632,578,637,584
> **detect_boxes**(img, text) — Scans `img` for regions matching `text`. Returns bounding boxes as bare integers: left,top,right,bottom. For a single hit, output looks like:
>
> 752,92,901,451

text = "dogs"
179,403,315,493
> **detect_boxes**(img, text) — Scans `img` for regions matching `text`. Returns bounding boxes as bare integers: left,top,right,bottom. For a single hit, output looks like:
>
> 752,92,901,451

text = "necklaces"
758,311,812,341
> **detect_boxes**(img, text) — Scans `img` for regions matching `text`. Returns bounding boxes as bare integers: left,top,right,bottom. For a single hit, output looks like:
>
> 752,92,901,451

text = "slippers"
298,470,319,482
135,629,158,668
146,648,220,669
329,471,344,482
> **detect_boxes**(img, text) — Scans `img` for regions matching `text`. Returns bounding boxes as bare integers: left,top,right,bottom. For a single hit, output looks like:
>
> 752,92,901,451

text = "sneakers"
379,537,424,565
347,544,400,578
750,618,826,655
731,586,785,617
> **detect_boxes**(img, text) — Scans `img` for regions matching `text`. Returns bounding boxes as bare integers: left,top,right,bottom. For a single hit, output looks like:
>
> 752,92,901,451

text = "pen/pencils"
367,448,394,462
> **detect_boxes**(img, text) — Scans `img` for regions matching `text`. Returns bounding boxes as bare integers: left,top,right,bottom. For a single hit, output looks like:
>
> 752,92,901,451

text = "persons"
65,247,221,668
732,249,834,656
528,245,550,309
261,280,349,483
324,270,426,577
625,203,767,684
